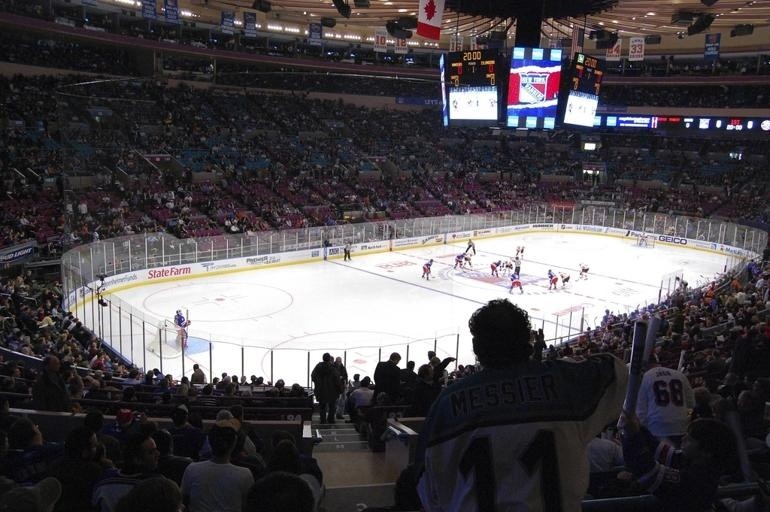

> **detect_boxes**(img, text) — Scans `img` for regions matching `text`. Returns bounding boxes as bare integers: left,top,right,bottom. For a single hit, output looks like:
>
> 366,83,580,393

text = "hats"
117,408,135,424
208,418,241,443
0,477,61,511
248,472,321,511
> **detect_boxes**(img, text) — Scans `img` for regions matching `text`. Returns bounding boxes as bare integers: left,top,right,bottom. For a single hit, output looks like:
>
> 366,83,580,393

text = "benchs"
0,362,412,452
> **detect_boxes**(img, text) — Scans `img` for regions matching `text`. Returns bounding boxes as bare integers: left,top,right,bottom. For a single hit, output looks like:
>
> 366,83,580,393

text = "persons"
0,1,770,512
